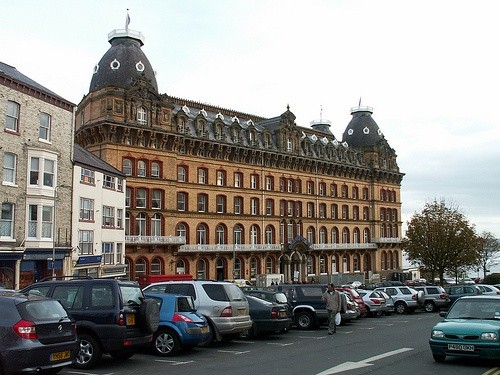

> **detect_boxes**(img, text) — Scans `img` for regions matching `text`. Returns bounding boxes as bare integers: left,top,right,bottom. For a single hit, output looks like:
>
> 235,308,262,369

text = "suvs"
19,276,155,370
267,284,367,331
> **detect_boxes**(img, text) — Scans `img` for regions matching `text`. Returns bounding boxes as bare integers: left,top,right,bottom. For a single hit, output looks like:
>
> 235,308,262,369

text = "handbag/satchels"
334,312,341,325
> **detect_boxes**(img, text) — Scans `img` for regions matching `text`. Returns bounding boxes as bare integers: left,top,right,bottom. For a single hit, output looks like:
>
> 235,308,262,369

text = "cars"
429,293,500,364
139,275,294,358
0,287,78,375
338,280,362,288
358,286,451,314
364,281,404,291
405,278,427,286
427,277,500,301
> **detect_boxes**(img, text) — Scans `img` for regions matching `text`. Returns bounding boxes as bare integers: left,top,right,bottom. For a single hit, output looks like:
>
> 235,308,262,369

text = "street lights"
53,185,72,281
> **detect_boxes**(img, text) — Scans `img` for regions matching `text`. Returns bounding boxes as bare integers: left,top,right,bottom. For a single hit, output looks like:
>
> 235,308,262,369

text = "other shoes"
328,331,333,335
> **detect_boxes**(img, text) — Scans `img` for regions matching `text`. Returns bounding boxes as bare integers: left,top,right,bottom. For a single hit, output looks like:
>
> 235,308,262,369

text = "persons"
322,283,341,335
